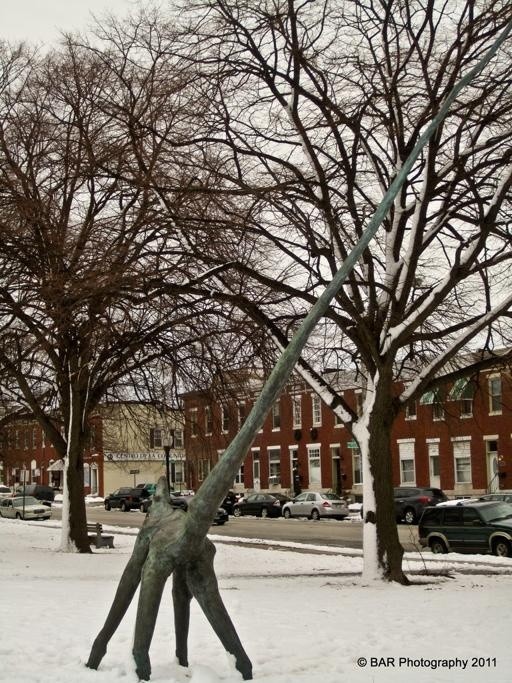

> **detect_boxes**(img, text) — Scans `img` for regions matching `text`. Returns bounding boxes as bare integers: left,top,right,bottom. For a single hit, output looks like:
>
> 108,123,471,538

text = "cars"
0,482,55,519
102,484,512,558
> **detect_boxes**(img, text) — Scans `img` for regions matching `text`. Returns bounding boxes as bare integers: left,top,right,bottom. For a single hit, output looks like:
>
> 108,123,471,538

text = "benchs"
86,522,115,549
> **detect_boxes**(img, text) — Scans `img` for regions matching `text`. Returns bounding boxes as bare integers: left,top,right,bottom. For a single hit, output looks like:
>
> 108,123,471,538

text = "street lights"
161,432,173,496
